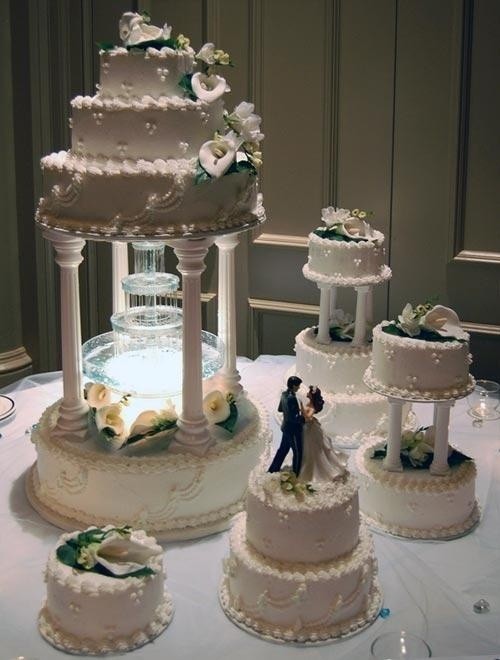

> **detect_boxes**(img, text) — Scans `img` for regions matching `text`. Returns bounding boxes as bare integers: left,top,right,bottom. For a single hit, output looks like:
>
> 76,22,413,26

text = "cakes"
218,376,384,647
37,524,175,654
274,322,417,449
27,381,273,529
354,425,480,544
365,302,476,400
303,206,393,285
33,12,266,238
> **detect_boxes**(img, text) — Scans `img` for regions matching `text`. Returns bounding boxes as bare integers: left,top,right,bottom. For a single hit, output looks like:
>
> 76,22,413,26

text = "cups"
369,630,432,660
466,380,500,421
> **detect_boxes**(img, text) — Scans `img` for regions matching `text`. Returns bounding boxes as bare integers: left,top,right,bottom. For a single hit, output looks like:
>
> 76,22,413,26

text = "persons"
300,385,350,488
268,375,304,477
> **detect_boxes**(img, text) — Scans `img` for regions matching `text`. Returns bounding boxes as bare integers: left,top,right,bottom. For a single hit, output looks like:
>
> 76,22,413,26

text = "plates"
0,394,17,423
